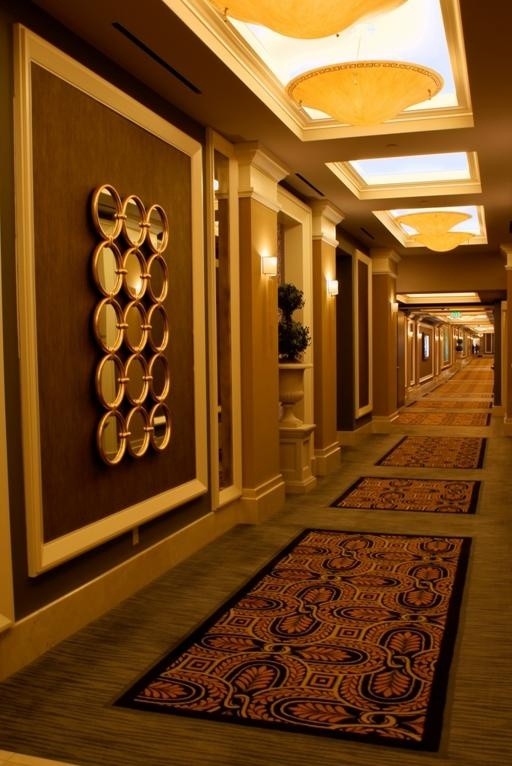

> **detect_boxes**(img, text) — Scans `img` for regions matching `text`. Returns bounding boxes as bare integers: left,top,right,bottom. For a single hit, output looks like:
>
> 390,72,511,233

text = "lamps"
370,205,491,253
396,291,497,335
327,280,339,295
325,151,484,200
262,255,277,277
161,0,475,143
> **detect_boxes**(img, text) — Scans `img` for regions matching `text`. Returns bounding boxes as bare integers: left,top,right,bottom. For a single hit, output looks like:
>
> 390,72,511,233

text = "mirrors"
90,183,175,466
208,127,243,512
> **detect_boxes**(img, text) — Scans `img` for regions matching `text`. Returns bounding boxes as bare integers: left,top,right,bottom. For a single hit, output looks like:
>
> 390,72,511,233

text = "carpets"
102,526,475,761
374,434,487,470
327,475,482,516
389,353,495,428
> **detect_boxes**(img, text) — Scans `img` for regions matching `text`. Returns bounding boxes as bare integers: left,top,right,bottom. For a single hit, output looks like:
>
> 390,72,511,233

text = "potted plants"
276,282,314,428
